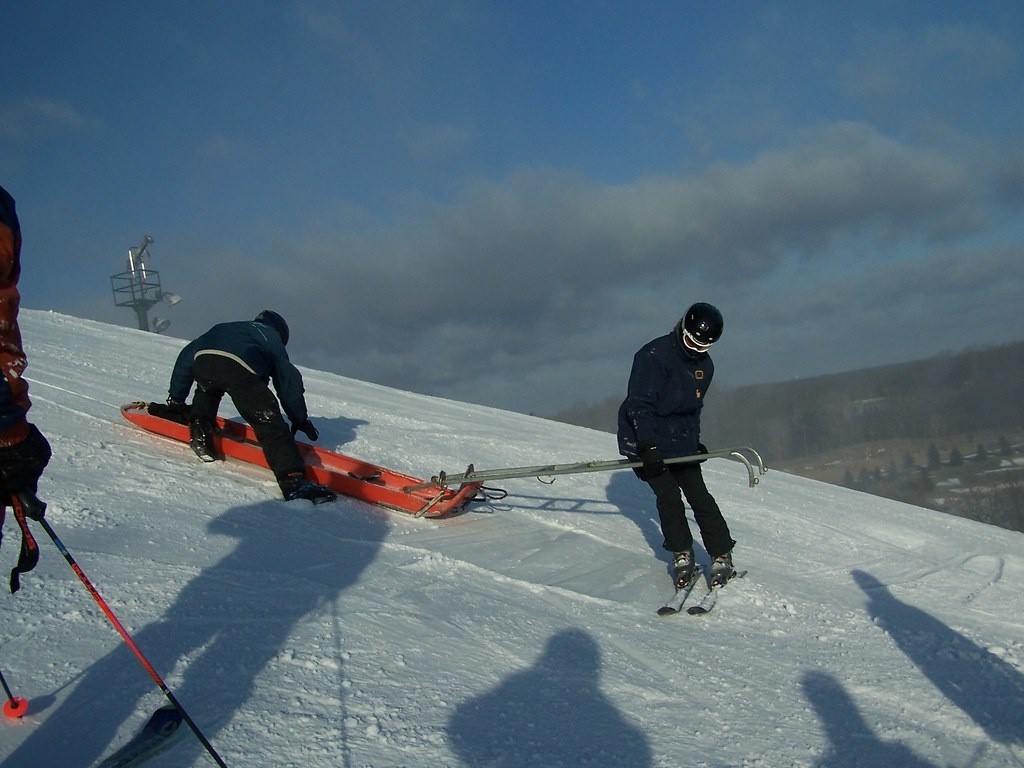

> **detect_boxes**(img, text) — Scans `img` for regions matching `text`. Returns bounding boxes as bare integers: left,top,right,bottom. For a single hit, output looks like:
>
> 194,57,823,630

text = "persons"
166,308,338,505
616,302,736,583
0,183,53,591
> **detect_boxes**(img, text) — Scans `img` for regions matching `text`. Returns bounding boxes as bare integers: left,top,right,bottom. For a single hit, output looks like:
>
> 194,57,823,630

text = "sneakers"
279,472,337,504
189,417,219,462
710,549,734,587
672,548,695,588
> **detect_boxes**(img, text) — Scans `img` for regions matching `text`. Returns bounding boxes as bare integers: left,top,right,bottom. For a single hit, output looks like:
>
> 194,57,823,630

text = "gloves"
291,418,319,441
697,443,708,463
0,424,52,505
167,396,186,407
640,441,667,477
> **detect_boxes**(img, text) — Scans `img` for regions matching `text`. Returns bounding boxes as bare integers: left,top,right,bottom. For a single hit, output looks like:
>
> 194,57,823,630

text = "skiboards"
656,563,734,616
96,704,184,768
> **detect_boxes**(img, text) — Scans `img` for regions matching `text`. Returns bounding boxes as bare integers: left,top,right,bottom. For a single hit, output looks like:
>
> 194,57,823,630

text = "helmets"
254,310,289,346
674,302,723,363
